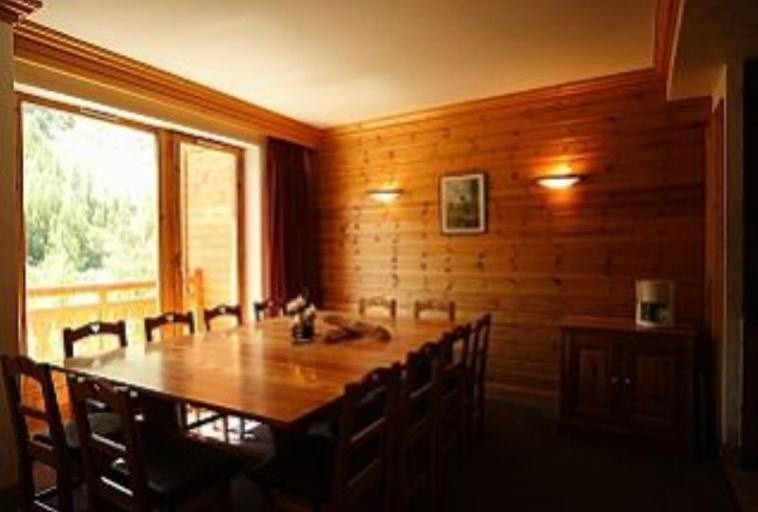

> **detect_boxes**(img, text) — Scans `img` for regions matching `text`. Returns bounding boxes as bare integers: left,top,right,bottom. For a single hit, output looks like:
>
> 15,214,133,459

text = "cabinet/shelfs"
557,314,711,442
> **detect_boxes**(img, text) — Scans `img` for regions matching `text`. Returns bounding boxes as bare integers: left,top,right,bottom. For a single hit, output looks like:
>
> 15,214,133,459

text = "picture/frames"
438,172,485,236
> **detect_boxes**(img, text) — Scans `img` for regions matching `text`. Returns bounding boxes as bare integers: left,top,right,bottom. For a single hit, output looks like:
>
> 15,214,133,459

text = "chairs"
303,360,403,499
254,296,285,321
415,299,456,322
358,297,396,319
63,320,127,415
401,341,443,465
203,304,242,332
467,313,493,431
0,354,120,512
144,310,194,342
439,321,470,450
65,372,207,512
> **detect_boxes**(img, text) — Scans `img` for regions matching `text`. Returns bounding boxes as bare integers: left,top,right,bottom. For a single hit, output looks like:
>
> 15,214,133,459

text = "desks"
50,312,469,459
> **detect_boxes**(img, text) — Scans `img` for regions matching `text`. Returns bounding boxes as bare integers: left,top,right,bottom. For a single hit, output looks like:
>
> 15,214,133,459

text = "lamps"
533,172,582,190
368,189,404,200
